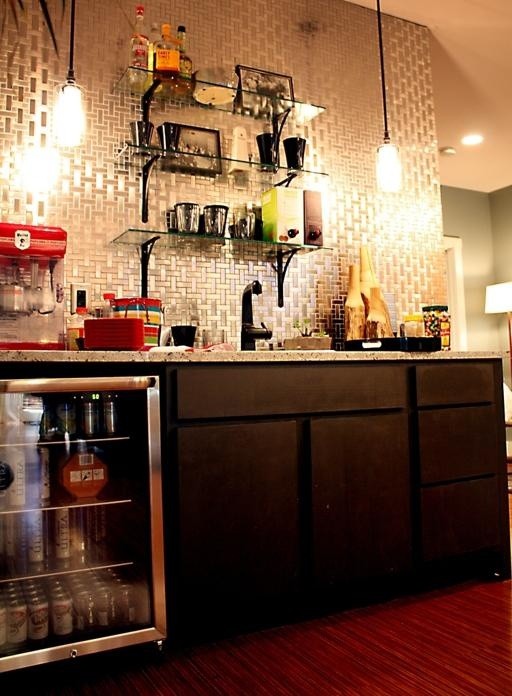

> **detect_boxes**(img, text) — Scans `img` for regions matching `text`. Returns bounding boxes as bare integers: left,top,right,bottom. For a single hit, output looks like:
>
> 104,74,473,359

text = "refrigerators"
0,374,169,675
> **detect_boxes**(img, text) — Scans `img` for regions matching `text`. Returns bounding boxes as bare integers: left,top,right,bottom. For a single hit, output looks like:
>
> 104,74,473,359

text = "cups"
232,202,255,239
130,120,154,157
283,137,306,168
157,122,181,152
171,325,197,347
256,133,278,172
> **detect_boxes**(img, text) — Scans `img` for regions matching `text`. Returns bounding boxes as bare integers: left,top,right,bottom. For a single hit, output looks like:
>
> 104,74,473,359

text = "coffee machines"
0,221,67,350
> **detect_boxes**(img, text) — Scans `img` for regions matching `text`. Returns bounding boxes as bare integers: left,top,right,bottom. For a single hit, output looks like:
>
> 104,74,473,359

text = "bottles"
127,5,149,71
177,25,193,79
100,293,115,318
153,22,181,73
67,307,93,350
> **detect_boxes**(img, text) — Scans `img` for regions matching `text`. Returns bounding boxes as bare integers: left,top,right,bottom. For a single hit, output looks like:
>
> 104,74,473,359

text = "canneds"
38,447,52,508
422,306,450,351
1,569,138,646
40,394,119,439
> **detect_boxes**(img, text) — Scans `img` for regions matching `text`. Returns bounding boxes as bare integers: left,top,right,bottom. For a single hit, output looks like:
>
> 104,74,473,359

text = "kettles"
166,202,200,233
203,205,229,237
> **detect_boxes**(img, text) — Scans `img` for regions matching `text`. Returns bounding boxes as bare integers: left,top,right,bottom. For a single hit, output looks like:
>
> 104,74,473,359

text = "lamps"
373,0,405,194
48,0,87,151
483,279,511,345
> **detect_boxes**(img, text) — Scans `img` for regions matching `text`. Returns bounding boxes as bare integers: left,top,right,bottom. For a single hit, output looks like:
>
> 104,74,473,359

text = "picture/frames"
163,120,223,176
236,64,296,113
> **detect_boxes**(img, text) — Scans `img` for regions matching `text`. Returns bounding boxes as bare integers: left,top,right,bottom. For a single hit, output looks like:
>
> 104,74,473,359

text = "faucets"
240,280,273,351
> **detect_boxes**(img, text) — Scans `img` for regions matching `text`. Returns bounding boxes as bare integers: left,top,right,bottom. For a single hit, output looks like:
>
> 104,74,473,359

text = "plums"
309,232,316,240
314,229,320,237
288,229,295,237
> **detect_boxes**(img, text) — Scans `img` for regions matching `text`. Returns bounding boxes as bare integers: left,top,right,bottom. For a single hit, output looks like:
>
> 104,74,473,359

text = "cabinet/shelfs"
108,64,335,308
413,361,511,598
0,349,174,693
168,361,415,648
